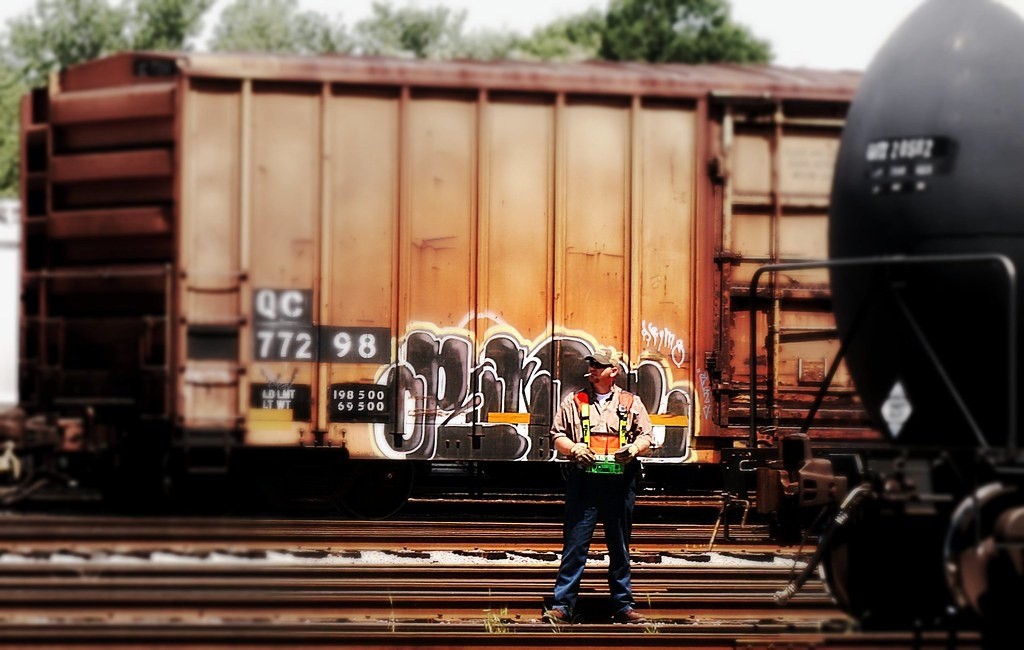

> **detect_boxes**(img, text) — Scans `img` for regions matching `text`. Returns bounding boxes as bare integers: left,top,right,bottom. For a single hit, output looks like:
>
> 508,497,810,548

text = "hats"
585,348,619,367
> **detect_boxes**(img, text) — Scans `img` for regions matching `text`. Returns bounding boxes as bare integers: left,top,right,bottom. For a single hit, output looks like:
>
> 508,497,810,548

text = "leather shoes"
542,610,569,625
614,610,646,623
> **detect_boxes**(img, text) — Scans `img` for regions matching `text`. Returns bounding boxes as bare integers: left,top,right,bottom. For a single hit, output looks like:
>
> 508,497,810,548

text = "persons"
541,348,654,624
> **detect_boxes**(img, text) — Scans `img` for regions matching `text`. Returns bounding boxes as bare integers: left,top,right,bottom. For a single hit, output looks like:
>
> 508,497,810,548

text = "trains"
0,49,891,507
703,1,1024,650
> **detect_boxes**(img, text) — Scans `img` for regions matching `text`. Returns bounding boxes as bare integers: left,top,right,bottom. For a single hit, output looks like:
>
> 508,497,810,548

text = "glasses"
588,360,609,368
